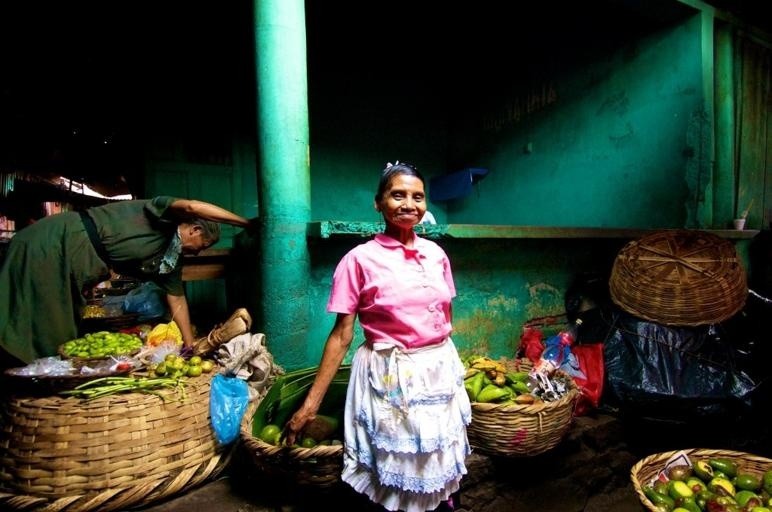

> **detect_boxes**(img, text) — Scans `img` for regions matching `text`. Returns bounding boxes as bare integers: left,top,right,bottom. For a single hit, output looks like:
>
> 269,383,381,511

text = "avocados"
645,459,772,512
259,415,342,448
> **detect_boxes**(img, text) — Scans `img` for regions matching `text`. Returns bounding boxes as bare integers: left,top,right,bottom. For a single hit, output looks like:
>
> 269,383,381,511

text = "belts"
78,208,116,270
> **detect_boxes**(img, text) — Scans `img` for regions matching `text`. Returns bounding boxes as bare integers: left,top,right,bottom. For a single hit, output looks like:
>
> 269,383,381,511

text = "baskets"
239,368,355,495
630,447,772,512
78,274,147,332
0,368,236,512
56,333,143,359
1,354,131,395
609,229,748,326
466,359,579,459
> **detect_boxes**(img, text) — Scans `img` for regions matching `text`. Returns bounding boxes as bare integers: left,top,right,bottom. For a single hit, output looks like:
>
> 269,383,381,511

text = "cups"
733,219,746,230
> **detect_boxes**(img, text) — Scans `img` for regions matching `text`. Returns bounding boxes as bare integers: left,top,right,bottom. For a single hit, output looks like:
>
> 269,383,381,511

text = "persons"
278,158,475,512
1,192,263,404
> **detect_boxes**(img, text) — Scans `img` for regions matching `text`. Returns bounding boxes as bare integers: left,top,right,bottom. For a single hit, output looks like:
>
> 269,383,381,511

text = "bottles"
523,316,584,392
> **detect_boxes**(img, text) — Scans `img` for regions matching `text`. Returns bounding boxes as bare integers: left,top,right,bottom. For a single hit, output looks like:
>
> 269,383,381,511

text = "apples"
148,353,213,380
